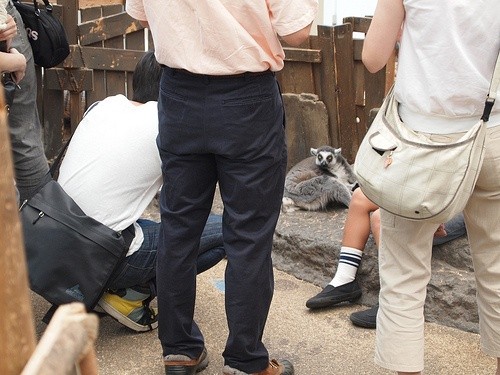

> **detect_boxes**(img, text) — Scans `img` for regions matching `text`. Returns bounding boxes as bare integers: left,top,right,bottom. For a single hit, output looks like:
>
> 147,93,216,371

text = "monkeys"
277,143,361,215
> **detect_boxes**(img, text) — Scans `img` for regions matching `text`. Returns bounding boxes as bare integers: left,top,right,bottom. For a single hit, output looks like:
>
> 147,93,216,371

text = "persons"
57,51,223,332
126,0,318,375
307,186,467,328
354,0,500,375
0,0,54,206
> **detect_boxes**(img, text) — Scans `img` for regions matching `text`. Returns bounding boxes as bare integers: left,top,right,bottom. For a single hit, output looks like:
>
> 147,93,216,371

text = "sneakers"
93,304,107,314
222,358,294,375
164,345,208,375
306,279,362,309
97,288,158,332
350,305,379,328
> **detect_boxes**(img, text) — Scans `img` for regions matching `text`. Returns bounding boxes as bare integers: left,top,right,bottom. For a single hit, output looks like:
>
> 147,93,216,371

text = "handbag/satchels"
1,71,21,115
354,83,488,223
12,0,70,69
18,178,129,315
